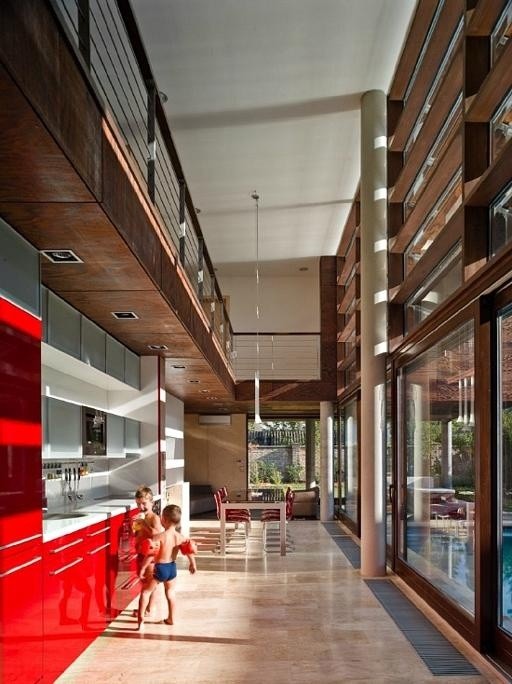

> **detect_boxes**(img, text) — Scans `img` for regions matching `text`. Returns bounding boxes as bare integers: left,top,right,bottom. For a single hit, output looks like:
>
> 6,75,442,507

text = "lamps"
249,193,269,431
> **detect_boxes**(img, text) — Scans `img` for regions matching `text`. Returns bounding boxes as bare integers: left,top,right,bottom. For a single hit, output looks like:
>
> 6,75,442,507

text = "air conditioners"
198,415,232,426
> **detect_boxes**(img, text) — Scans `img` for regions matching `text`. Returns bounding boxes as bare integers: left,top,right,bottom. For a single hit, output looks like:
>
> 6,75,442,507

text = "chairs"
292,487,319,521
190,484,217,516
212,485,294,554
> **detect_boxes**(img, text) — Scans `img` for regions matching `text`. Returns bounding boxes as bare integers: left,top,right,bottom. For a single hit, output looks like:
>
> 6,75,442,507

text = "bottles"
80,463,89,476
44,463,62,479
132,512,146,532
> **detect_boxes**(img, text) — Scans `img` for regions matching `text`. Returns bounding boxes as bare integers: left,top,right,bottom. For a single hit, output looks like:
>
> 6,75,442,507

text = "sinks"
41,513,88,520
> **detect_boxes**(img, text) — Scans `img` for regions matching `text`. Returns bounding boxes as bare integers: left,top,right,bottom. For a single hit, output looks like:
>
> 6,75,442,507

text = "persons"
135,504,197,631
131,485,163,617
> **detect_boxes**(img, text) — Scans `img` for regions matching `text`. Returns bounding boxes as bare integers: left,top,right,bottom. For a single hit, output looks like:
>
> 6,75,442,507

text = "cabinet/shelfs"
0,509,159,684
0,295,43,557
0,217,141,390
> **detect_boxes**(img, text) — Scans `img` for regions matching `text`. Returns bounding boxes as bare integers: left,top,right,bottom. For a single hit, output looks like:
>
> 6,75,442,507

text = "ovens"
82,408,106,456
